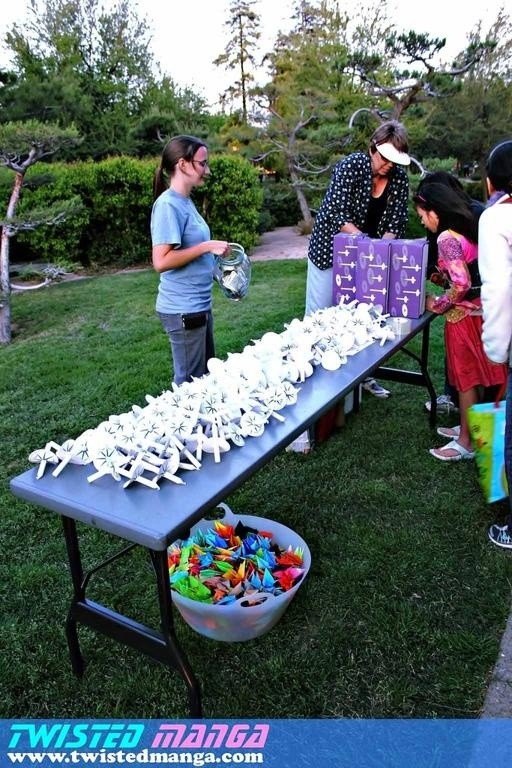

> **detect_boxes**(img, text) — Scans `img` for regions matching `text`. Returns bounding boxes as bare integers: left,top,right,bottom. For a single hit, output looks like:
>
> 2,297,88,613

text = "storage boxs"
332,234,361,304
389,240,430,319
356,238,389,313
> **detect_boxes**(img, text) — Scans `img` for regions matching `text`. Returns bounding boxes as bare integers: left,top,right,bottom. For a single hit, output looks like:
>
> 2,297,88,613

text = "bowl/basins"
168,501,312,643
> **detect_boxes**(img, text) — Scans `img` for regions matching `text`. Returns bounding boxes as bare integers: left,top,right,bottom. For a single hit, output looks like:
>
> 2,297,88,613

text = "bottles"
211,242,253,301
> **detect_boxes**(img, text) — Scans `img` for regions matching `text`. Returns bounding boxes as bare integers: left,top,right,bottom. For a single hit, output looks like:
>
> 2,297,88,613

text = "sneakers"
488,524,512,549
361,378,392,398
424,394,459,415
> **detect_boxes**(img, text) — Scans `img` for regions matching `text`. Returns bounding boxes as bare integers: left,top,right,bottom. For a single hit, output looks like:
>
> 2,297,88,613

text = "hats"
372,138,411,166
486,139,512,176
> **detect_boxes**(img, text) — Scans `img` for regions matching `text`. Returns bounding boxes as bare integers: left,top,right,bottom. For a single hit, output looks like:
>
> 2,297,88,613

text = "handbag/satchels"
464,363,510,505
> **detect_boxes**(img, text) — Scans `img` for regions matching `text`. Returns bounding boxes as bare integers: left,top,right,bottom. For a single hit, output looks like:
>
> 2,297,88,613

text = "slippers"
429,440,476,461
437,424,461,441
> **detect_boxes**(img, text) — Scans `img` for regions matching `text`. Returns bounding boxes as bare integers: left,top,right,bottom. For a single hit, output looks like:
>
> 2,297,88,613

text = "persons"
303,120,410,400
150,135,232,391
412,142,512,550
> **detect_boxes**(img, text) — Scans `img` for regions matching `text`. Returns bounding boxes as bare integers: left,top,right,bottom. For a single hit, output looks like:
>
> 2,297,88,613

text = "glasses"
192,159,209,167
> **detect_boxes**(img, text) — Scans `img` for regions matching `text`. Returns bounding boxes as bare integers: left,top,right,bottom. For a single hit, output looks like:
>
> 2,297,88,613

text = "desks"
11,304,439,720
